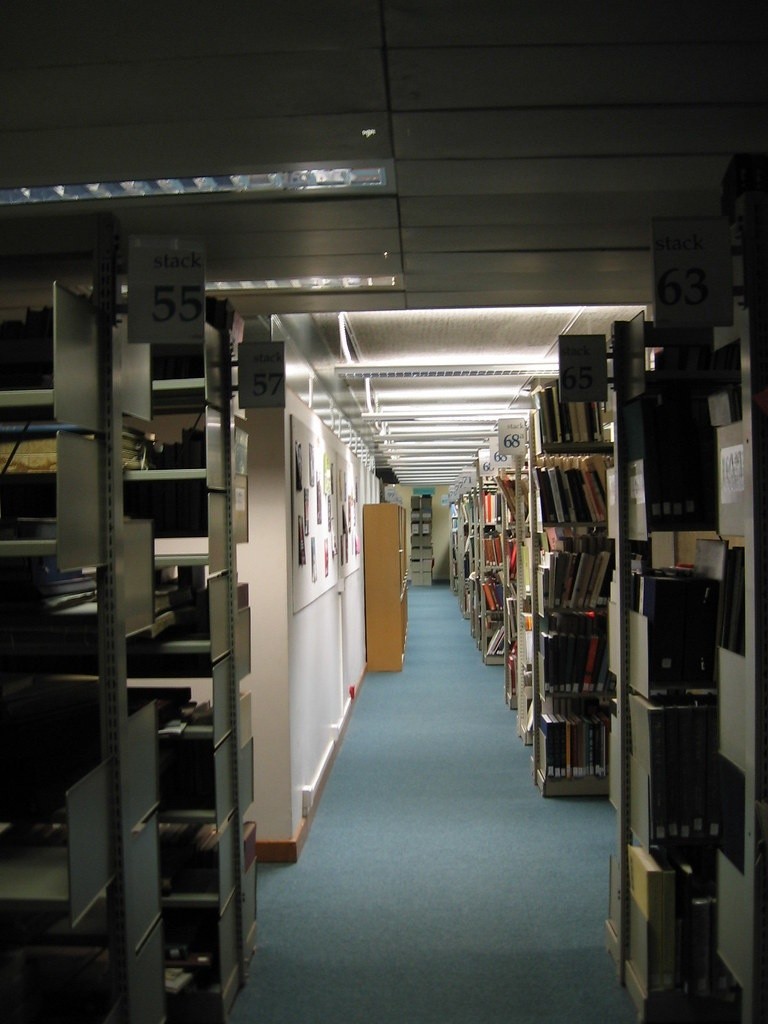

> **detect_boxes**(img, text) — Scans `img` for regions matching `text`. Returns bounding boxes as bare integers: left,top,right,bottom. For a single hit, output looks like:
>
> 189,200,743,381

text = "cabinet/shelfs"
362,503,409,672
450,185,768,1023
1,211,257,1024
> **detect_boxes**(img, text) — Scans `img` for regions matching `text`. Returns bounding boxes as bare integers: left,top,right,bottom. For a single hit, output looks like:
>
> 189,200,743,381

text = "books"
0,296,234,1024
453,334,746,998
